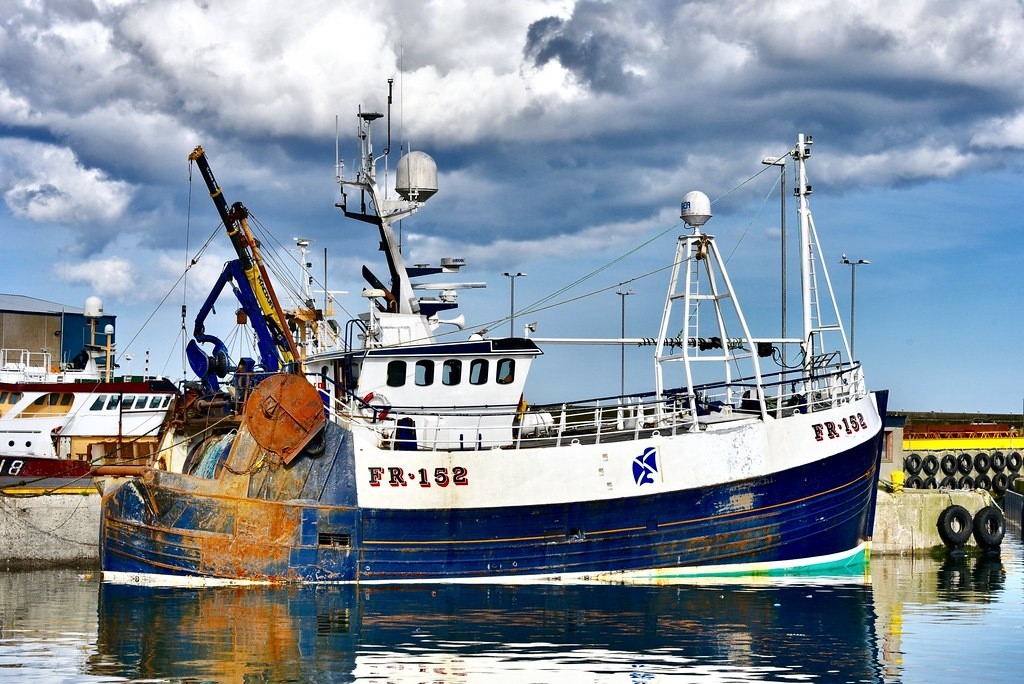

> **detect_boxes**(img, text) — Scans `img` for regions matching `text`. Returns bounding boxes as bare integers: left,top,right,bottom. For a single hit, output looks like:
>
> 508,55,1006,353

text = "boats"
0,297,180,481
92,75,895,601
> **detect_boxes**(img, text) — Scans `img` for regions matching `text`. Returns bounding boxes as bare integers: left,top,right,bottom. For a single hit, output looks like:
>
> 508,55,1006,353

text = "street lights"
760,155,788,405
834,251,871,360
501,271,528,338
615,289,637,404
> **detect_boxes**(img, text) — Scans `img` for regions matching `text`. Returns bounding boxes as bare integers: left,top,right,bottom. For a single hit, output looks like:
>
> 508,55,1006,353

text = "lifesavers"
902,451,1023,494
937,560,1008,593
938,505,1007,548
358,392,391,424
